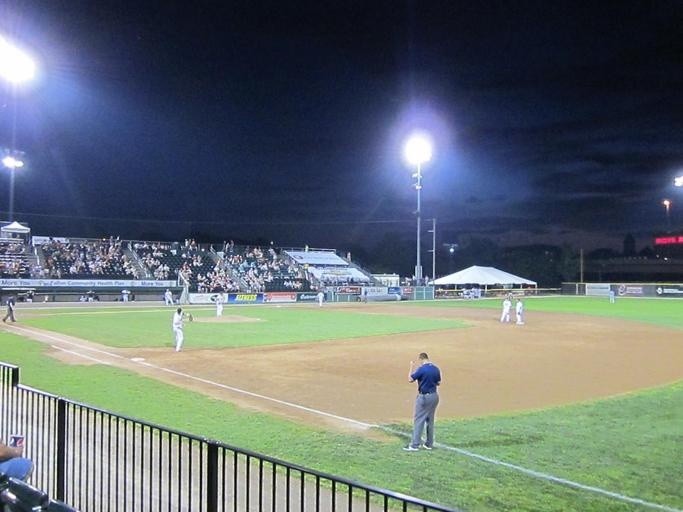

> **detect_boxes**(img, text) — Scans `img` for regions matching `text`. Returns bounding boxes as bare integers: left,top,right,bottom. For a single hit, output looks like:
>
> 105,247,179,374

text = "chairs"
0,473,77,512
0,236,314,293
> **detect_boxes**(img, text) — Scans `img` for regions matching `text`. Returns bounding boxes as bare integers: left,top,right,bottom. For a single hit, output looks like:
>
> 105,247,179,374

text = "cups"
9,435,24,447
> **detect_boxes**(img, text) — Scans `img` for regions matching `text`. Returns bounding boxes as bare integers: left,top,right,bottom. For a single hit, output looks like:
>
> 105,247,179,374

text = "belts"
419,390,435,394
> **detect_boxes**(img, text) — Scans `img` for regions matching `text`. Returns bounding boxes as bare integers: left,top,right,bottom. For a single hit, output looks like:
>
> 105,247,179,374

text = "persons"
172,308,190,352
500,291,523,324
0,235,482,321
403,353,440,450
1,441,32,492
609,290,615,304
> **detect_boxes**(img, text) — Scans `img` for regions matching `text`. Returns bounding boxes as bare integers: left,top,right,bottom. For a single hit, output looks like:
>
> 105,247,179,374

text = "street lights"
661,198,671,235
0,48,35,223
404,134,433,288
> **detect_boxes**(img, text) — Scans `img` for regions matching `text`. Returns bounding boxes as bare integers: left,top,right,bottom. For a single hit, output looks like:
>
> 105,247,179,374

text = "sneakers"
403,443,419,451
422,442,432,450
